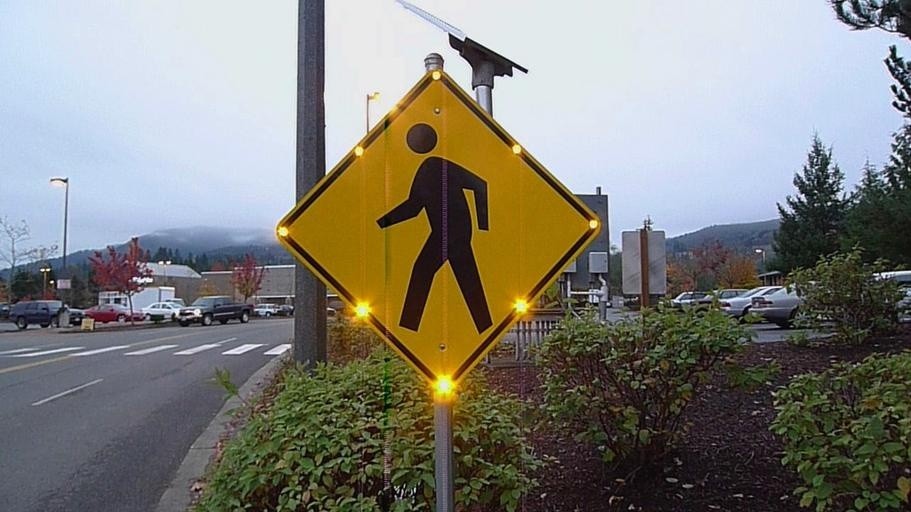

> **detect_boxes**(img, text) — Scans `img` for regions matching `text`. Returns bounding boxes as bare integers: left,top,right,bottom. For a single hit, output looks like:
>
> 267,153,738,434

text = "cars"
624,270,911,327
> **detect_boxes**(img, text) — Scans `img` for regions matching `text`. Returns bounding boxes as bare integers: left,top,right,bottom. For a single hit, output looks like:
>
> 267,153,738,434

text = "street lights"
40,268,51,299
366,90,381,135
754,248,767,286
51,178,68,268
158,260,172,286
